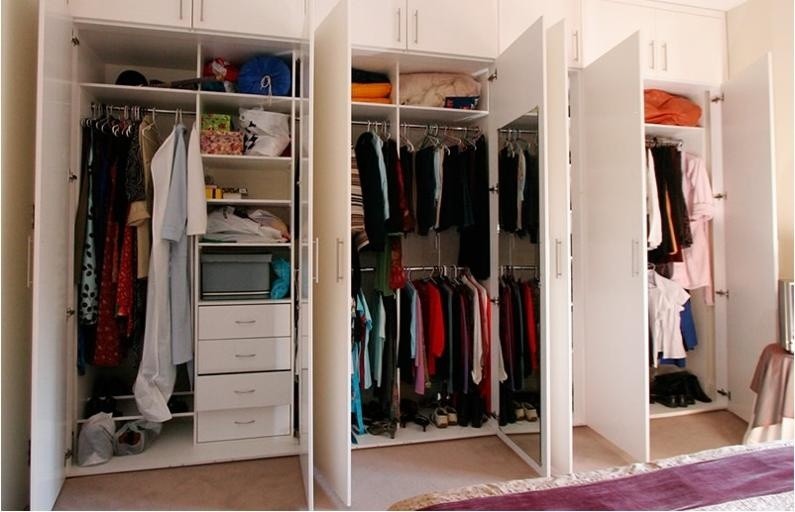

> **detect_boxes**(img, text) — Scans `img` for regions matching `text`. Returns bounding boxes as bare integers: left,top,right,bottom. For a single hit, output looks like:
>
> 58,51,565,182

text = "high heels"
367,422,397,439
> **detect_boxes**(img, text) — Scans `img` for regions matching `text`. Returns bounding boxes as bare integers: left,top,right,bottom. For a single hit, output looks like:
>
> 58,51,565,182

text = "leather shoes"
656,379,713,408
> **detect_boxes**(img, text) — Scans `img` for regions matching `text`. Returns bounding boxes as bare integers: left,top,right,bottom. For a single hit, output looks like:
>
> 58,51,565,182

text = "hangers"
78,103,185,148
366,122,479,155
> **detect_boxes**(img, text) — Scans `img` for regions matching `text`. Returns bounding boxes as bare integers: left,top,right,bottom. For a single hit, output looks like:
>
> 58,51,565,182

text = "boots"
460,395,489,428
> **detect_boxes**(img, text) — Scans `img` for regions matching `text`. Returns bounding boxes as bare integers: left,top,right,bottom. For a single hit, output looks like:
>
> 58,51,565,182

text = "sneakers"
516,400,537,423
431,404,457,428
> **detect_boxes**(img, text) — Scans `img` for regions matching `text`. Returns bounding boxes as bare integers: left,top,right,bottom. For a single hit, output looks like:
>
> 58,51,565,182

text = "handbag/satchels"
238,107,291,157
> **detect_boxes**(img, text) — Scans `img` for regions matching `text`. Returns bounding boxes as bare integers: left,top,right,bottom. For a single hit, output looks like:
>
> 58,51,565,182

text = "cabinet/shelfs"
29,0,782,511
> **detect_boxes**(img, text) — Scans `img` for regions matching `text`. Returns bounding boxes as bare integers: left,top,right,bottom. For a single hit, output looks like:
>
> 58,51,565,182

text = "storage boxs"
198,251,273,300
